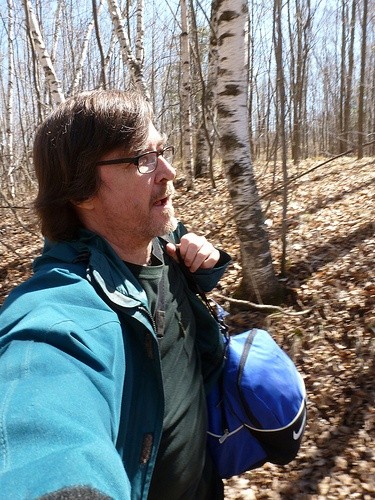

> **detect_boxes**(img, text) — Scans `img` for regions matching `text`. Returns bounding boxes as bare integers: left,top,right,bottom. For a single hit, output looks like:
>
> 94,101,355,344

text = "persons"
0,89,233,499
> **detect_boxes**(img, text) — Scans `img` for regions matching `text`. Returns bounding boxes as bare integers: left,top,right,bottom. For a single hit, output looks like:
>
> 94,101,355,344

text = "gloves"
201,325,308,479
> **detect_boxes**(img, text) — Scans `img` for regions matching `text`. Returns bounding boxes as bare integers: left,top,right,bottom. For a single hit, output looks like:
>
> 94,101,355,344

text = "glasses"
96,144,175,172
